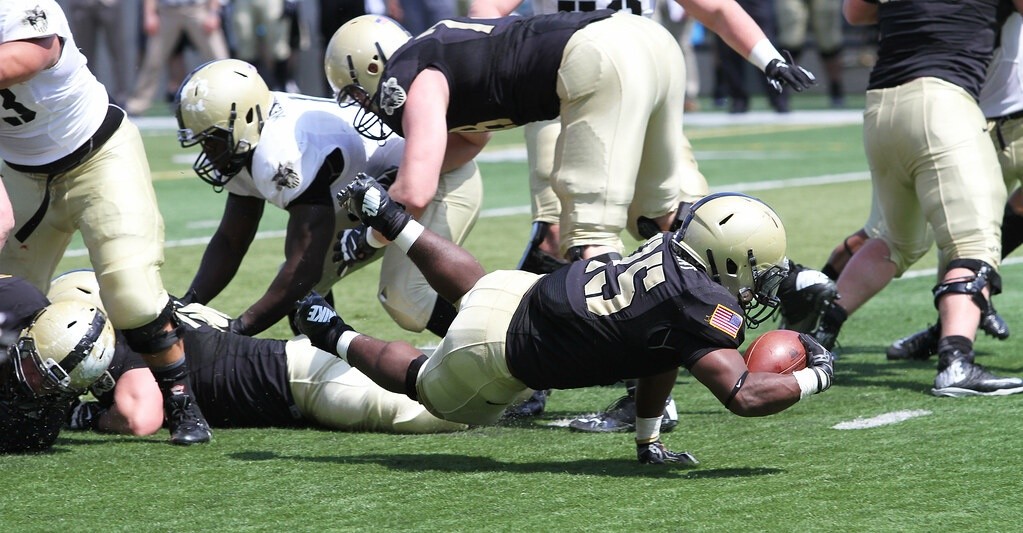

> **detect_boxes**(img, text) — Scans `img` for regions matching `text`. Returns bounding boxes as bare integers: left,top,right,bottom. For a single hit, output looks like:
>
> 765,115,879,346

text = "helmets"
672,192,787,332
15,299,117,394
46,270,101,300
177,58,275,194
323,13,413,147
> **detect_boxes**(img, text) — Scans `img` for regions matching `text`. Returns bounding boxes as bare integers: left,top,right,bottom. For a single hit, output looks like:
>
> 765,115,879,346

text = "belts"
3,93,124,243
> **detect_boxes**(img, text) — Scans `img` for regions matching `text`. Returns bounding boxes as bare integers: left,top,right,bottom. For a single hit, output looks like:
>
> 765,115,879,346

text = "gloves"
759,55,816,91
177,304,244,336
800,331,835,391
638,437,699,466
333,226,387,279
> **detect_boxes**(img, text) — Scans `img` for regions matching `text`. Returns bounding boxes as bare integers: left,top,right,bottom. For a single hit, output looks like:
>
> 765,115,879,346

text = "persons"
174,57,543,417
301,0,535,96
293,171,834,466
464,0,818,418
784,0,1023,397
0,0,469,456
325,13,842,432
652,0,846,113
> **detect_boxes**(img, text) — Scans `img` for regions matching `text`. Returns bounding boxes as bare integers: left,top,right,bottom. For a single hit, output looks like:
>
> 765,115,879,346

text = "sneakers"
167,394,212,443
980,304,1010,340
337,172,390,223
293,290,339,353
887,327,942,360
930,349,1023,397
504,387,549,420
570,390,679,434
803,300,847,353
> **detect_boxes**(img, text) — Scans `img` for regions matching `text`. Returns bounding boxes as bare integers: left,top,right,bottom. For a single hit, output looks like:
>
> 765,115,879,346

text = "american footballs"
743,330,808,374
